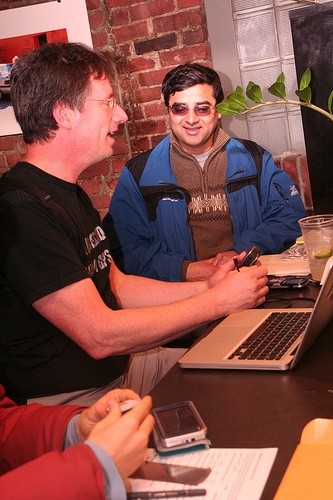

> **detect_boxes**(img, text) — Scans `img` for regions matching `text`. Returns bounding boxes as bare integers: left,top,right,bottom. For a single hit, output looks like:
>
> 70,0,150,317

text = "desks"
125,286,333,500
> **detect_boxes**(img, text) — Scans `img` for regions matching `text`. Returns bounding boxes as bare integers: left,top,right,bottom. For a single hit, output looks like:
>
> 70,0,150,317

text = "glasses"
167,102,216,116
85,96,116,111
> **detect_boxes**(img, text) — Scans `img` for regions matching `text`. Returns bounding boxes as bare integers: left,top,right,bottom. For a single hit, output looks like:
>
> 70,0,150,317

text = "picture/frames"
0,0,96,137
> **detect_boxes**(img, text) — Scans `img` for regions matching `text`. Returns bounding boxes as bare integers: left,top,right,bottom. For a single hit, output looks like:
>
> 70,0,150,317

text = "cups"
298,214,333,282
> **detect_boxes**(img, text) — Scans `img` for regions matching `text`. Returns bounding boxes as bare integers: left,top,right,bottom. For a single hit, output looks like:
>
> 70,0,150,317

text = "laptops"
179,262,333,371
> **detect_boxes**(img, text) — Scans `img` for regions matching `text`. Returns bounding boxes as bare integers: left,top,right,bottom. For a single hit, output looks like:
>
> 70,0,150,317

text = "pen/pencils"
233,256,239,272
127,489,206,500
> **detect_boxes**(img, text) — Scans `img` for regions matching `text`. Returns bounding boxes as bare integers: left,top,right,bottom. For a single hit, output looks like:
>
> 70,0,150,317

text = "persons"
0,42,269,406
0,384,155,500
108,62,308,283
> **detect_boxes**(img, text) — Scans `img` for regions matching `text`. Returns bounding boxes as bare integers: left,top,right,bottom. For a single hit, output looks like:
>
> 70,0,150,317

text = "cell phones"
268,277,308,290
150,401,206,447
